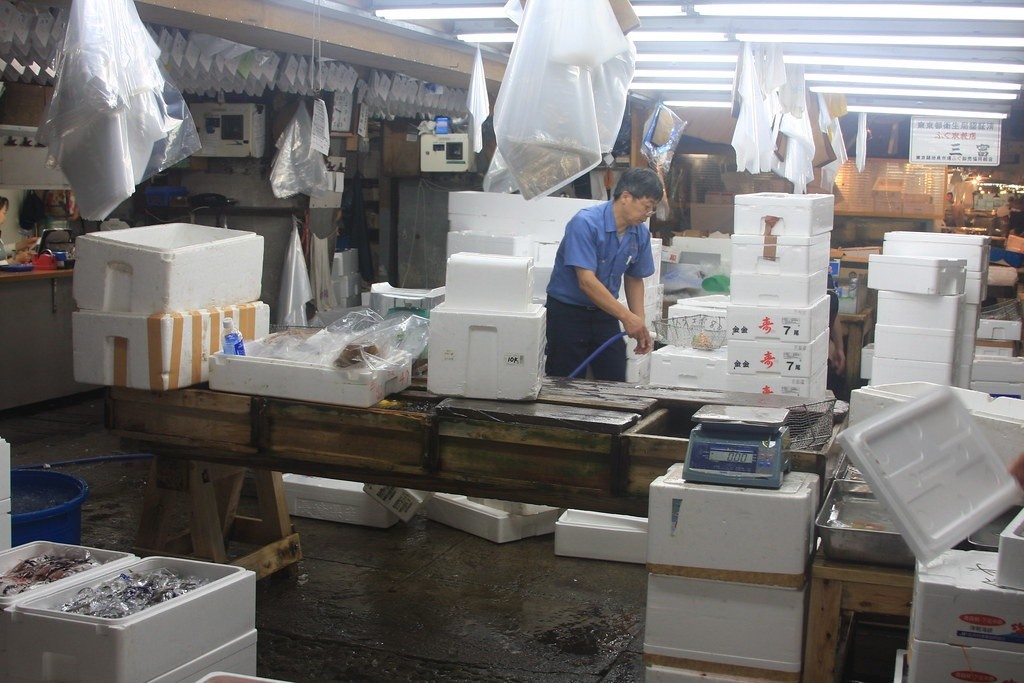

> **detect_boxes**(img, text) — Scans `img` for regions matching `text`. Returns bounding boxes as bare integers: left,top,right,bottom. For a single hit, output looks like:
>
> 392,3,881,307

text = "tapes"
40,249,53,255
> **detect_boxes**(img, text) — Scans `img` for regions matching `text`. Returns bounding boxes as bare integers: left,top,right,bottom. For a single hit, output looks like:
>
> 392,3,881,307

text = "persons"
545,166,663,384
0,196,39,264
824,289,849,401
945,193,956,226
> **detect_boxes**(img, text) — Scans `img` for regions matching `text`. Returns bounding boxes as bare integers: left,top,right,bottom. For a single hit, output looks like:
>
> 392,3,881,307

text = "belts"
549,296,602,311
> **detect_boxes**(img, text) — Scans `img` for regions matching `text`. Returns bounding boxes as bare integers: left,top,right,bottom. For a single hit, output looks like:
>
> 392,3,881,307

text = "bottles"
220,317,245,356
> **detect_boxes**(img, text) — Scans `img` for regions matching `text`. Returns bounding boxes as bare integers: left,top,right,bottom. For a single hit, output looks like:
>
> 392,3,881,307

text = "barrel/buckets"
10,468,89,549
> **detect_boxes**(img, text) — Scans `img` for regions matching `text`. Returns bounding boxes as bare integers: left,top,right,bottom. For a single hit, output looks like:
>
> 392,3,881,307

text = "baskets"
652,315,727,350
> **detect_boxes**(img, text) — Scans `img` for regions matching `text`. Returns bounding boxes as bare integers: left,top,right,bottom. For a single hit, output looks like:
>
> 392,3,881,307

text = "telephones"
191,193,238,207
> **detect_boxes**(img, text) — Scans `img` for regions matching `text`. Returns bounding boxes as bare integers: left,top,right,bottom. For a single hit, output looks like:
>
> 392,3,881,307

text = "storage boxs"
170,196,189,207
0,189,1024,683
3,81,54,126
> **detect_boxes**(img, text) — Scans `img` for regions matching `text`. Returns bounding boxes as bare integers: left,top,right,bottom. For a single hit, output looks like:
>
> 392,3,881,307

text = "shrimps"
0,552,91,595
65,567,201,618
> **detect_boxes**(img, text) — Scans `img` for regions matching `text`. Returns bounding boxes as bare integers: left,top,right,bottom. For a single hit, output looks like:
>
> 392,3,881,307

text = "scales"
682,405,790,489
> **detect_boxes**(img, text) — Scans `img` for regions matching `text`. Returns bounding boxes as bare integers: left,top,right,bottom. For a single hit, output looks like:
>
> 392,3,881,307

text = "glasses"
634,196,656,216
